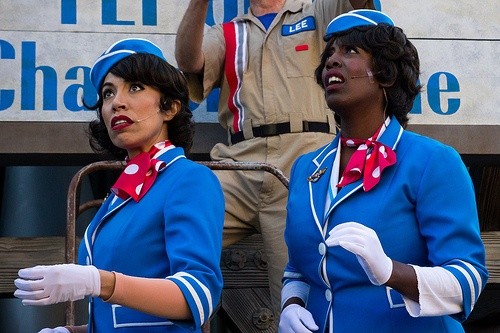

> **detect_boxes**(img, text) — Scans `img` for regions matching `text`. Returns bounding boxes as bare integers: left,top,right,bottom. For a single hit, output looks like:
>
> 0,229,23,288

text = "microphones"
351,70,391,82
137,97,171,122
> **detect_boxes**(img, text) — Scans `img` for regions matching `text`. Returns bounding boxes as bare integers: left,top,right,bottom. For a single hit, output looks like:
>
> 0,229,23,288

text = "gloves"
278,304,319,333
13,263,101,305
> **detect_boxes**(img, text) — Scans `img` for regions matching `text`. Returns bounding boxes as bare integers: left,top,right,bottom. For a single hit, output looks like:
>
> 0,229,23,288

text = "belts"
324,221,394,286
231,121,329,143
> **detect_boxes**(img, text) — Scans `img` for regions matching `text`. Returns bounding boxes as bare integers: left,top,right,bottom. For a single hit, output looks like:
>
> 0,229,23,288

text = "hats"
324,9,395,38
89,38,167,95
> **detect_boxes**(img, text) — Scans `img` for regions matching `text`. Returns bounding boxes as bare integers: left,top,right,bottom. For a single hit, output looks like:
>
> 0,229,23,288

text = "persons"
175,0,376,333
14,37,225,333
277,9,490,333
